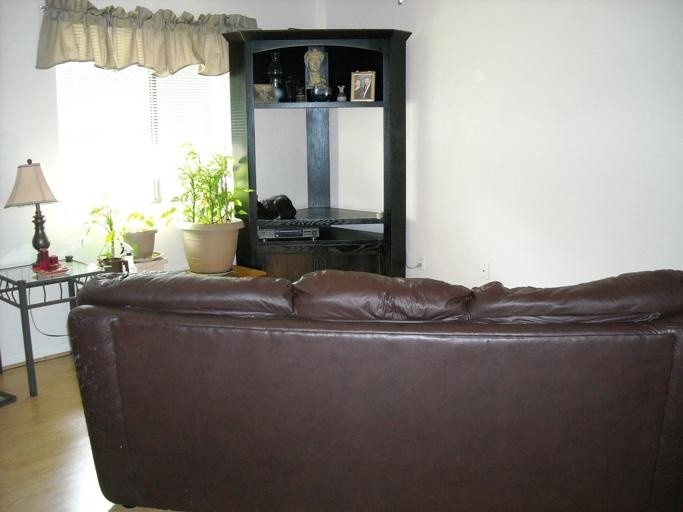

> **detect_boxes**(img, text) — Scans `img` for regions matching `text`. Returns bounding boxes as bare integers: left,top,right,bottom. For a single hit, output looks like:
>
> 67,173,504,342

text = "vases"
122,228,163,263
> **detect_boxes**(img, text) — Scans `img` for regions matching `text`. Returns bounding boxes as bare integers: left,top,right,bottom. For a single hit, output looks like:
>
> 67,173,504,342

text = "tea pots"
99,258,129,272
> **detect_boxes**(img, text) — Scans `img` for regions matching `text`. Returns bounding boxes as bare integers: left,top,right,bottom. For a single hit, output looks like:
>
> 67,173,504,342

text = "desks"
0,256,112,396
176,264,268,278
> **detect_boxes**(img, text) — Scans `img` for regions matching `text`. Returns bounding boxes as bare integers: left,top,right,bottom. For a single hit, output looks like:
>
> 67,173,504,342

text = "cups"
310,86,321,102
321,86,333,102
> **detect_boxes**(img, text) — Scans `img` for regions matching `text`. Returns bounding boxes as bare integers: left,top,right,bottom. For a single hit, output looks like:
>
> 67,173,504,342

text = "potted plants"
84,202,132,275
161,150,256,276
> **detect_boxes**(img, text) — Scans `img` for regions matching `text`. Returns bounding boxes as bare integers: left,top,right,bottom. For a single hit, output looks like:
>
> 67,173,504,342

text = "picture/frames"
350,71,377,103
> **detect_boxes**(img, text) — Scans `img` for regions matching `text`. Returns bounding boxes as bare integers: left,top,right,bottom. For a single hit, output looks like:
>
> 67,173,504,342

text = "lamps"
3,158,58,268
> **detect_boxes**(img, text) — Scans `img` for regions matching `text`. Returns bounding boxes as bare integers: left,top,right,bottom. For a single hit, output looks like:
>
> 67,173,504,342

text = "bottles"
268,52,286,102
284,79,296,102
296,85,304,102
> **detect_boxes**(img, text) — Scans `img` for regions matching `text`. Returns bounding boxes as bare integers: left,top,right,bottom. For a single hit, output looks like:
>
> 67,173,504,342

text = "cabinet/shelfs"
220,27,412,284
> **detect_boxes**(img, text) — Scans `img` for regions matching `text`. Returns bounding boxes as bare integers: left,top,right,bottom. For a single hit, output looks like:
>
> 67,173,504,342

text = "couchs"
67,269,683,512
